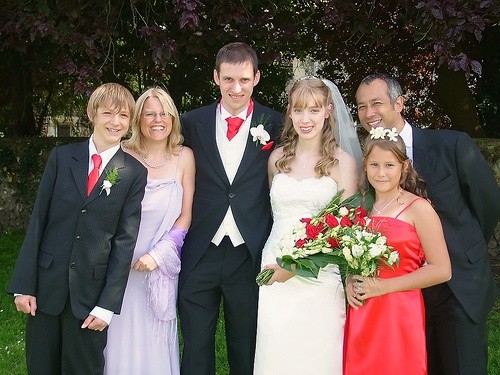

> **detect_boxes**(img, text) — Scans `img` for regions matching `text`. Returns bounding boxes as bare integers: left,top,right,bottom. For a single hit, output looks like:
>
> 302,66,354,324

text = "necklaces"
298,145,305,168
140,154,169,168
373,189,402,214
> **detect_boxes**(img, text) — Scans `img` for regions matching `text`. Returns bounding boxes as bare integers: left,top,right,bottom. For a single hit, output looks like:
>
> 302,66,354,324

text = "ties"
87,154,102,197
220,98,253,141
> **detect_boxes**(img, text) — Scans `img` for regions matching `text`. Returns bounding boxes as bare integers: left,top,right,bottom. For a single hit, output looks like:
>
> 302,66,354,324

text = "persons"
342,126,451,375
356,75,499,375
103,88,196,375
253,77,364,375
177,42,287,375
7,83,148,375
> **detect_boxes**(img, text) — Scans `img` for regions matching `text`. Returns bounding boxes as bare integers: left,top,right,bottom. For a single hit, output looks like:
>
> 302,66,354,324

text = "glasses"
141,111,171,119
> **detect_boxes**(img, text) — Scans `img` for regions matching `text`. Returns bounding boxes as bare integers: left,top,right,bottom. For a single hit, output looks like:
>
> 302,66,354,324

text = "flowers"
254,189,400,306
99,165,121,197
370,126,387,140
249,113,273,148
385,127,399,142
